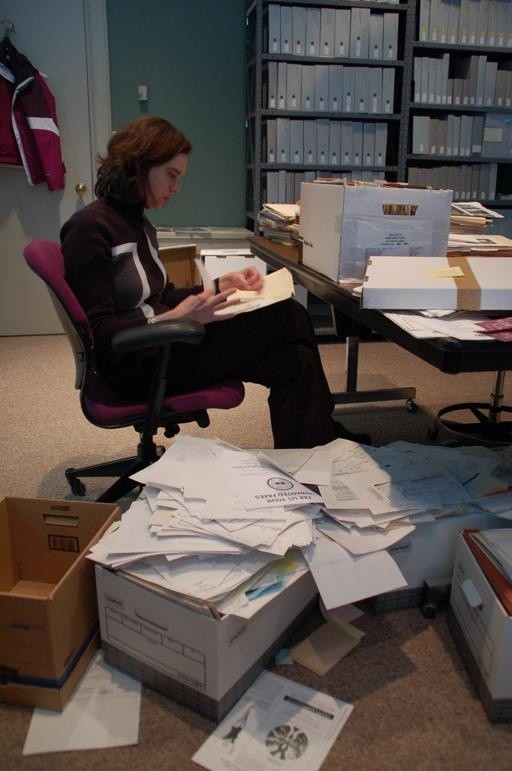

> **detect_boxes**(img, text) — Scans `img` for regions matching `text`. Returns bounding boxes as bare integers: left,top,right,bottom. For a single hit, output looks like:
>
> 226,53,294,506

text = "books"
409,2,512,200
260,178,511,253
212,265,295,319
263,4,398,205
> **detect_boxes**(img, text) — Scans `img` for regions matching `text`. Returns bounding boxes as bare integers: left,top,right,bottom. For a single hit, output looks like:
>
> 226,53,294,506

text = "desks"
244,233,512,450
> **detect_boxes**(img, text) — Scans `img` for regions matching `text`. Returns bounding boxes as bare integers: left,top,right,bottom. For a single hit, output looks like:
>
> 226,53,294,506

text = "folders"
407,162,498,199
262,61,396,115
411,112,511,158
264,1,401,61
259,170,386,206
416,2,511,47
265,117,387,163
413,52,512,108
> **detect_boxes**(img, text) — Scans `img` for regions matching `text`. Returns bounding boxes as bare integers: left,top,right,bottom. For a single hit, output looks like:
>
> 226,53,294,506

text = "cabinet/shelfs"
244,1,512,235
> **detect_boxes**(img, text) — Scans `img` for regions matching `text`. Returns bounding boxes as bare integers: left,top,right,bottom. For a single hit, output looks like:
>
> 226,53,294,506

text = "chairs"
21,236,247,506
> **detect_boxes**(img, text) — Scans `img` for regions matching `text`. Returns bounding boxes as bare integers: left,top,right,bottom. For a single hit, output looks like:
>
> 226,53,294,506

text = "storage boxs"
299,177,453,285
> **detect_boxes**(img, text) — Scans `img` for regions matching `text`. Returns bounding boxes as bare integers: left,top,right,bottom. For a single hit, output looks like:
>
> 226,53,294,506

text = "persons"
57,114,374,449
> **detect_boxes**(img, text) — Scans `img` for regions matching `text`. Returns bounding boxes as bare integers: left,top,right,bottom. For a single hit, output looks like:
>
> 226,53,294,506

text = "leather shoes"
332,419,371,445
